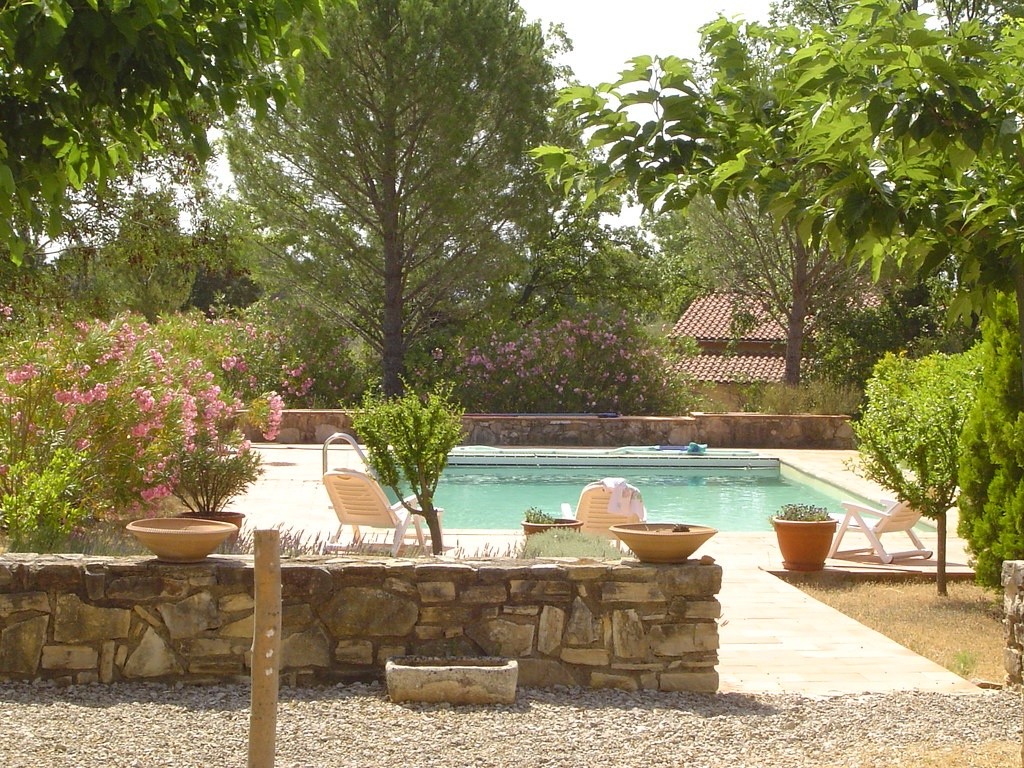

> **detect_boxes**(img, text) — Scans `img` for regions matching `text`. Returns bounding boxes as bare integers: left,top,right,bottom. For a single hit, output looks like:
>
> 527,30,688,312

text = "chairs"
326,472,442,558
560,477,646,545
829,491,933,564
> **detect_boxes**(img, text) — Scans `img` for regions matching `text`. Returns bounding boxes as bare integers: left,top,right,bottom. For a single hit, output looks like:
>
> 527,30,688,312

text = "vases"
384,655,518,706
126,517,237,563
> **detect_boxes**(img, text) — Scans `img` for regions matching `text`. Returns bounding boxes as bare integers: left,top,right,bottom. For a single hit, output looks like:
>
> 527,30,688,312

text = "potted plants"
771,501,833,571
616,523,718,563
522,508,581,540
170,452,264,551
